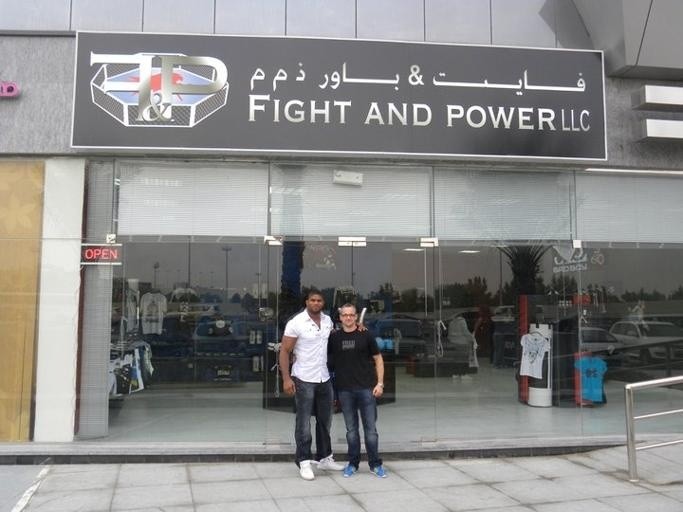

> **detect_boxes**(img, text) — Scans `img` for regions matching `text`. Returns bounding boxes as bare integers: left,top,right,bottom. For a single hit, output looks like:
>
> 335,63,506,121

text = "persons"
447,312,476,381
326,303,388,478
279,291,368,480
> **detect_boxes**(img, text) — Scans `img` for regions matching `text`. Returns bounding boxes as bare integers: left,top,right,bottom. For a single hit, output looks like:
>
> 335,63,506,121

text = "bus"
629,313,683,328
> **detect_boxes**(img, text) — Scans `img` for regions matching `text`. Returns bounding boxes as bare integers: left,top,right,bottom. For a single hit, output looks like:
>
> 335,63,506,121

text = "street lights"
221,247,232,301
255,271,263,309
152,261,160,289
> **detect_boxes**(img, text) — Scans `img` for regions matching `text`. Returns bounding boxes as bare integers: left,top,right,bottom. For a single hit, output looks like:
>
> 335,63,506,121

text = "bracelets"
378,383,384,388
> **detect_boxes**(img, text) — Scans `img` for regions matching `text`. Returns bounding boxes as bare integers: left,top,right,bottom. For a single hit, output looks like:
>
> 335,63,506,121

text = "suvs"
441,310,496,337
193,320,264,352
608,321,683,366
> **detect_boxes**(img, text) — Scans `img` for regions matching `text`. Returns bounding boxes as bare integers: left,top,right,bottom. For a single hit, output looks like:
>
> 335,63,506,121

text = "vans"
492,303,544,321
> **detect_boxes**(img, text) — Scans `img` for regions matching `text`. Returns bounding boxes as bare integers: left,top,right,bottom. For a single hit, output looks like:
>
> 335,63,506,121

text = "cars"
377,313,435,337
581,327,627,367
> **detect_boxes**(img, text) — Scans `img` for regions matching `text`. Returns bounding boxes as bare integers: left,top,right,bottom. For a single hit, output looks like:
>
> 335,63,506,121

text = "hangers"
529,328,543,337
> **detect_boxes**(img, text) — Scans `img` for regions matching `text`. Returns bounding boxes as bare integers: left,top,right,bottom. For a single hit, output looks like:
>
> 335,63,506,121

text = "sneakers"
317,455,355,476
370,466,385,476
299,459,314,480
